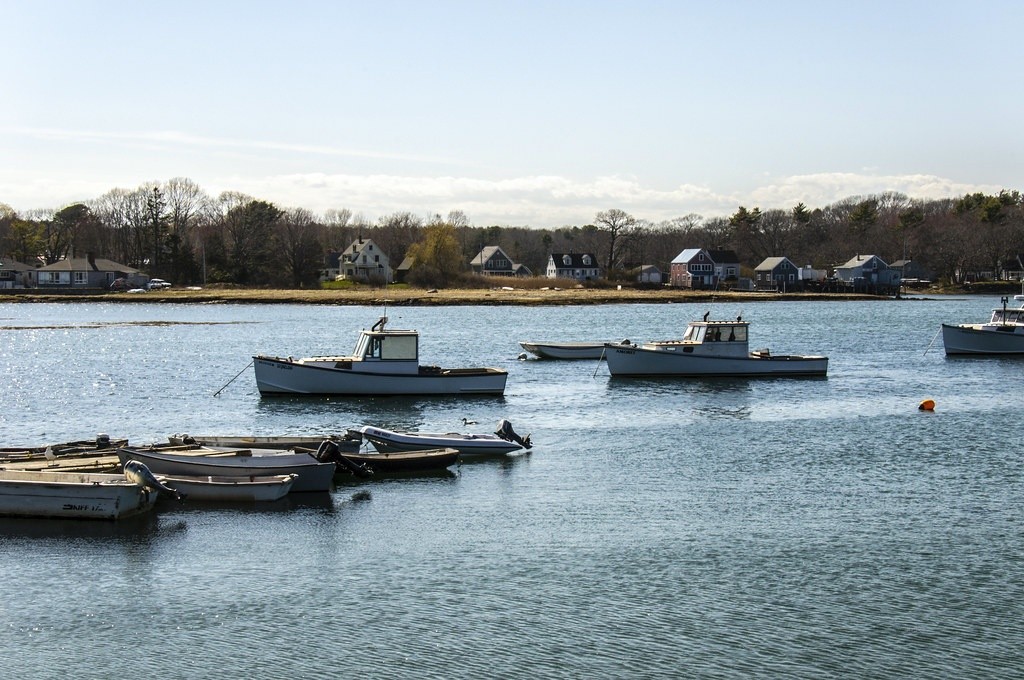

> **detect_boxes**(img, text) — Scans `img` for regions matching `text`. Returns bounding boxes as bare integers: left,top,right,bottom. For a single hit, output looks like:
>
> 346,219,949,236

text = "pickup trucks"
147,278,172,289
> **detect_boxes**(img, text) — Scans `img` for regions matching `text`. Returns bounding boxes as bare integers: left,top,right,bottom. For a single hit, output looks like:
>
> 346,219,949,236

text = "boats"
941,280,1024,355
0,467,165,521
150,471,298,501
116,448,338,492
251,249,509,396
519,342,617,360
361,419,534,456
603,274,829,376
0,430,460,476
